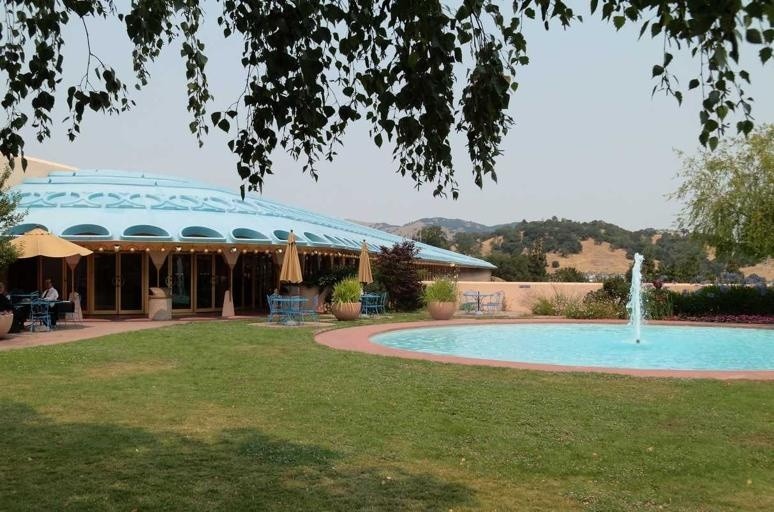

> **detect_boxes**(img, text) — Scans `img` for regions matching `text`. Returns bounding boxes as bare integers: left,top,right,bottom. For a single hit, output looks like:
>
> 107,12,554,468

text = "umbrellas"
2,226,93,326
279,228,303,319
358,239,373,313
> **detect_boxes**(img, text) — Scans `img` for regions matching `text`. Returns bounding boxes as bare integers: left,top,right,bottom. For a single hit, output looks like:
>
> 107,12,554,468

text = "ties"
44,291,48,299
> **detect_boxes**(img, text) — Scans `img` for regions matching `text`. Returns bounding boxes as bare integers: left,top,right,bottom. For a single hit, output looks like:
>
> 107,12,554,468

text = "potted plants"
421,279,457,319
330,279,362,320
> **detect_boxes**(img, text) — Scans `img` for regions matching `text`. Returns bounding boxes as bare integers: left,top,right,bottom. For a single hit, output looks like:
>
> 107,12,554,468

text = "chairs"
10,291,83,331
268,294,320,323
463,291,503,317
360,291,387,317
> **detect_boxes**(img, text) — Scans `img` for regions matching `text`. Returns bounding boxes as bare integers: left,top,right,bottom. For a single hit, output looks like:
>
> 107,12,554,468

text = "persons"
270,287,283,317
42,276,58,328
0,282,21,333
